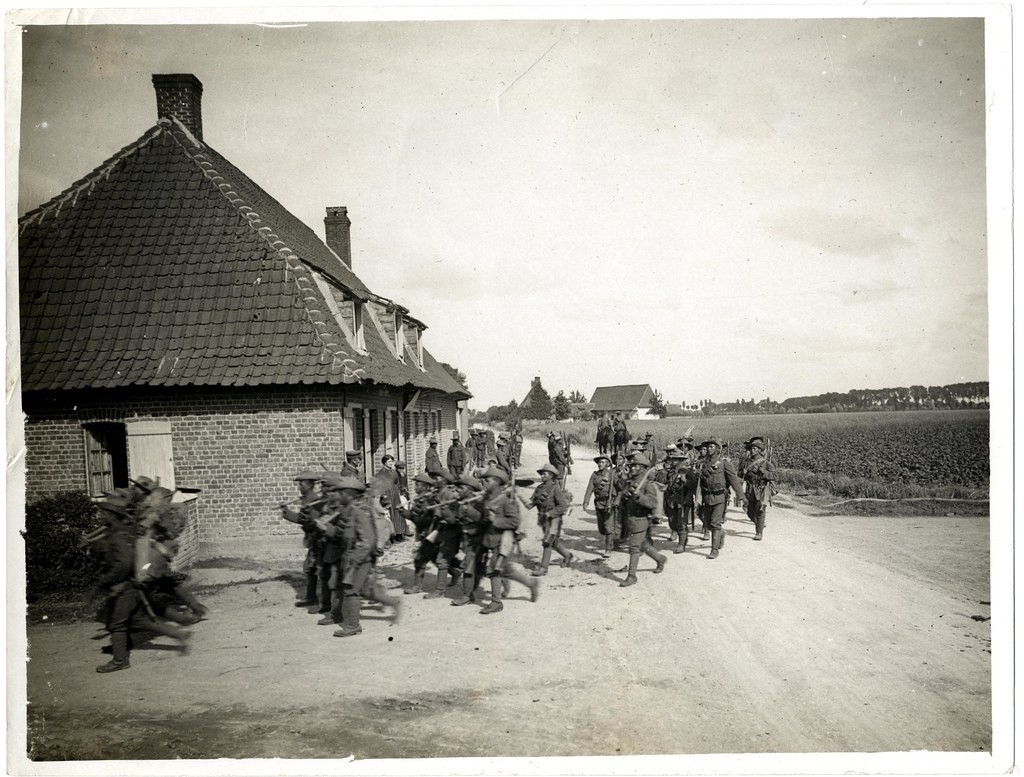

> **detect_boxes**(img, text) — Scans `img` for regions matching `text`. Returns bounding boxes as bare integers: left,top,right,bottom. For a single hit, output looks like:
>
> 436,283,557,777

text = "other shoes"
620,574,637,589
383,530,414,549
533,567,549,575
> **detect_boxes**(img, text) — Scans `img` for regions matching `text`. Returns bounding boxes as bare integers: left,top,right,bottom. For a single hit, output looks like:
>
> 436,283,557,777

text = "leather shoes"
511,462,521,469
603,530,630,558
559,552,573,567
295,592,401,637
667,526,725,558
97,596,208,672
654,554,667,573
754,532,764,540
404,576,540,613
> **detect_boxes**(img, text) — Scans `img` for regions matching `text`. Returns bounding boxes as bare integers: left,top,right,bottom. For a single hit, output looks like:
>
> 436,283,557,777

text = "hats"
292,468,367,494
599,409,622,416
427,430,523,446
593,431,765,464
411,465,508,491
99,476,155,521
548,429,565,443
537,462,559,478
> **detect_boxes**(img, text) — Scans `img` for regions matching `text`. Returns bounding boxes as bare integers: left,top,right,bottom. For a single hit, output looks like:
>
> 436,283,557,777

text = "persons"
88,476,209,673
283,415,778,637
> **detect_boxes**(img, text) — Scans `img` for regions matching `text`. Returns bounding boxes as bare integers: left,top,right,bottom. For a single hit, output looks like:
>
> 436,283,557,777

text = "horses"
597,425,631,456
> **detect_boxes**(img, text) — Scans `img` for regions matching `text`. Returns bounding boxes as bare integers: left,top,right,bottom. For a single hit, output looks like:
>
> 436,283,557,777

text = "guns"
541,427,577,554
390,479,519,517
68,482,199,563
268,485,390,535
599,429,776,535
